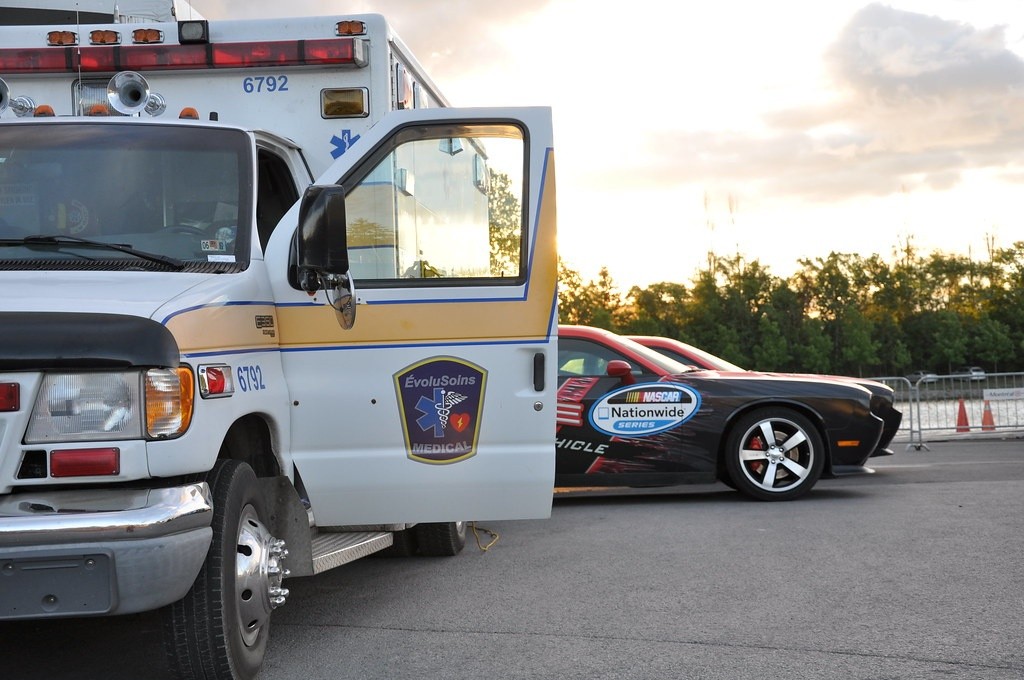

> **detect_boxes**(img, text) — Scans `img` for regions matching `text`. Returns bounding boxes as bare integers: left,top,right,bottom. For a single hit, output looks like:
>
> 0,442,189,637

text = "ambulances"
1,2,567,679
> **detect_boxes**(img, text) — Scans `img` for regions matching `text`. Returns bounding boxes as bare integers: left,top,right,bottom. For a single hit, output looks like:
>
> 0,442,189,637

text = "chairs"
181,151,283,252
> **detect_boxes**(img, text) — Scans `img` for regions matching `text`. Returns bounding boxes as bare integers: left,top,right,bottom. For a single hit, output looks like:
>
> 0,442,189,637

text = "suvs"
950,367,987,382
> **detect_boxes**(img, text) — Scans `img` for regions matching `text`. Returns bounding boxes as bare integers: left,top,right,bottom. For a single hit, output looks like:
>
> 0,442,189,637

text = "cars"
615,335,902,473
902,370,938,384
557,322,882,501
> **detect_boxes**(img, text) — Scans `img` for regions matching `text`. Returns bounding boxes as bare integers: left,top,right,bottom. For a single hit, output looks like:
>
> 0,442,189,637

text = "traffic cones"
954,399,972,433
979,398,997,431
